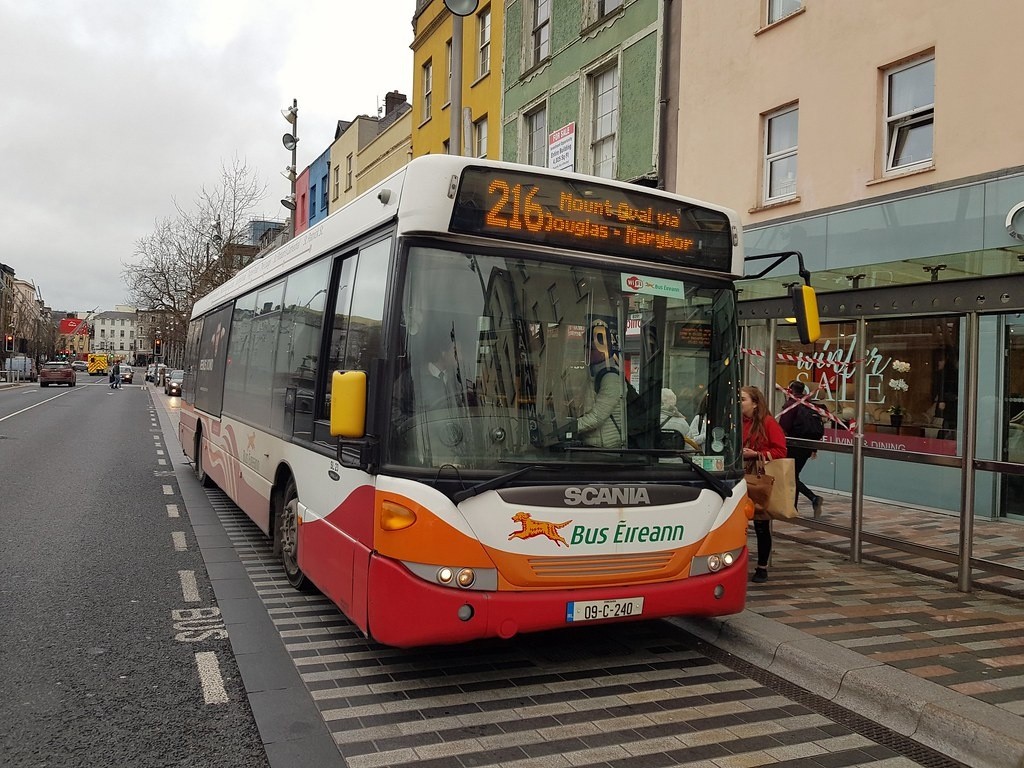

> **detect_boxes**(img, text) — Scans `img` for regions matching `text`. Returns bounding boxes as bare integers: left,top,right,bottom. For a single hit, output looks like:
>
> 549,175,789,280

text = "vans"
6,357,38,382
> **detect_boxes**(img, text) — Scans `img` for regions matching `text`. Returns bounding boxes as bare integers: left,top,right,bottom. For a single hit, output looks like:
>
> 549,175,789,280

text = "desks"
871,423,925,435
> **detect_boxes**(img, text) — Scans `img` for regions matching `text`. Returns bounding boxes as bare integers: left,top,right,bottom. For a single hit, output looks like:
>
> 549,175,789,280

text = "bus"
178,151,823,653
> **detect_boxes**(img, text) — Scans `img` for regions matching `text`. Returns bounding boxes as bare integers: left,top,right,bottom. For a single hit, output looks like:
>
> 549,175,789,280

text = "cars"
71,360,88,372
108,363,134,384
287,388,331,412
145,363,177,386
164,370,185,397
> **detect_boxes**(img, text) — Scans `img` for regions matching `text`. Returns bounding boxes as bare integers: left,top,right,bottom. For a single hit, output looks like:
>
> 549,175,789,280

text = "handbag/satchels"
750,452,798,519
744,451,776,521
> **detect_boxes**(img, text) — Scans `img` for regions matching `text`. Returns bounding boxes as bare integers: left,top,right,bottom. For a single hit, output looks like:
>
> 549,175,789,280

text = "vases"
892,388,904,406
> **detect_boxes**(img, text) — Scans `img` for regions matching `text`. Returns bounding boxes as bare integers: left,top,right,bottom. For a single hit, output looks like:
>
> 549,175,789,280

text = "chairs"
920,418,944,439
365,359,403,437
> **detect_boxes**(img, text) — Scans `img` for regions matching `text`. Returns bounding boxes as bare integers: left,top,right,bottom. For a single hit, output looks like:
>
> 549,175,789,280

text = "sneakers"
752,567,768,583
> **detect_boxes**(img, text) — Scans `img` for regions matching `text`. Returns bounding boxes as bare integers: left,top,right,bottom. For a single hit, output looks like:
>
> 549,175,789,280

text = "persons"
779,380,823,518
658,388,700,464
391,337,481,430
558,325,628,450
741,386,788,583
107,360,124,390
132,352,136,366
929,353,958,440
684,392,705,456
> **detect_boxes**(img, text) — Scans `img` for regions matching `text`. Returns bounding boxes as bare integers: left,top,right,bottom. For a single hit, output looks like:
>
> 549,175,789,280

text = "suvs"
40,361,77,387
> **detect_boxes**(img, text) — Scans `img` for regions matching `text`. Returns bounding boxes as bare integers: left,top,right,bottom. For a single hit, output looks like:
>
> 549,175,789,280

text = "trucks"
88,353,108,376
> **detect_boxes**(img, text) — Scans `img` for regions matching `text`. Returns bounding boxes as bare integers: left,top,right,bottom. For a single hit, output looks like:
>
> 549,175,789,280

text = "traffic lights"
6,335,14,352
73,350,76,357
154,339,162,356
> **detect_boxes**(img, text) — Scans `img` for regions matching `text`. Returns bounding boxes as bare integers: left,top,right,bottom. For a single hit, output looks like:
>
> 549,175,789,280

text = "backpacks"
789,398,824,440
595,367,661,455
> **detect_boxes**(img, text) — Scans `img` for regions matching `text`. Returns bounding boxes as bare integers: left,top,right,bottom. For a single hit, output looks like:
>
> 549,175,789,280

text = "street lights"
211,213,224,285
281,99,300,241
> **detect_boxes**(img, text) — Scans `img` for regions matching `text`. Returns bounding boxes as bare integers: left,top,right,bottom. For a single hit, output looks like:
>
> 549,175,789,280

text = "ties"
438,372,445,380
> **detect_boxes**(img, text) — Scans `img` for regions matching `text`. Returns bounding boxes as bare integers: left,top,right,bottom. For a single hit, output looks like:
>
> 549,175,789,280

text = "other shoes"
812,497,824,520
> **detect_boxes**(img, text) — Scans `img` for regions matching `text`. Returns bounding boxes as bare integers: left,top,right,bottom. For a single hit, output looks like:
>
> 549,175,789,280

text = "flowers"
889,359,911,392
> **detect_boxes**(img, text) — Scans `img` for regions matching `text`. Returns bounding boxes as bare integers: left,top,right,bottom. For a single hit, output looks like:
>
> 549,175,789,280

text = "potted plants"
886,406,906,426
